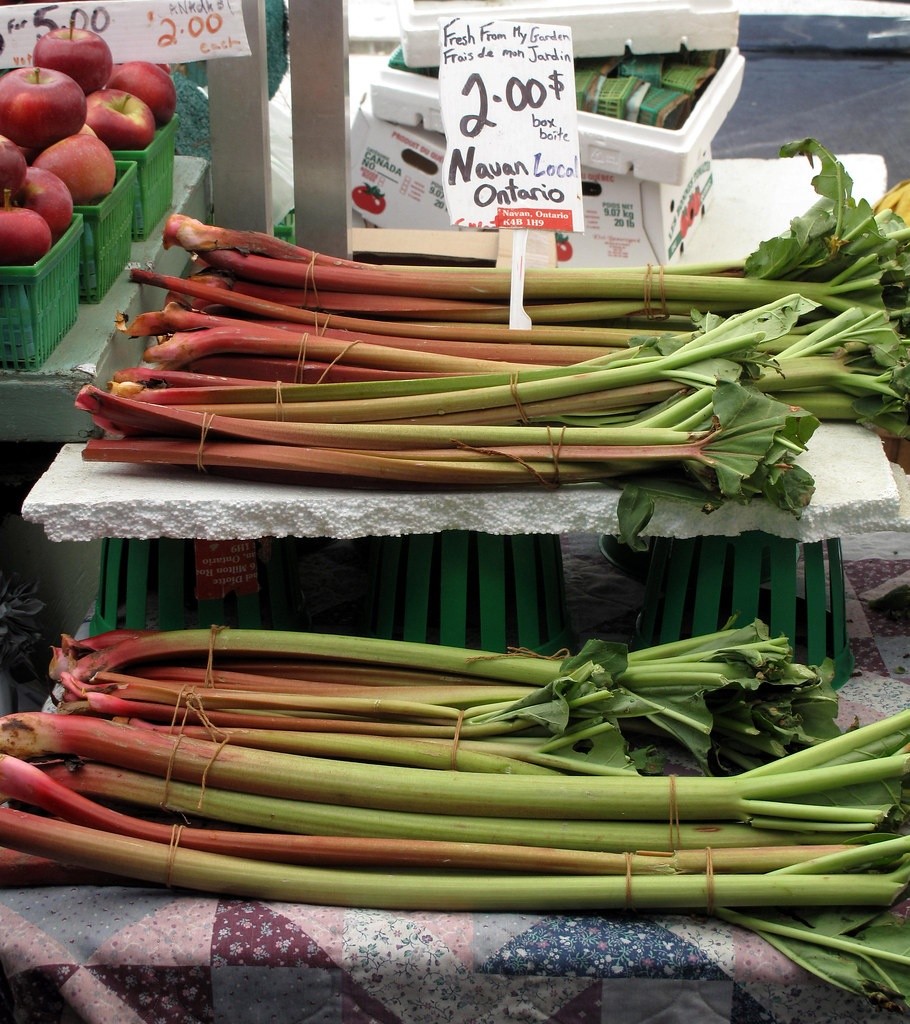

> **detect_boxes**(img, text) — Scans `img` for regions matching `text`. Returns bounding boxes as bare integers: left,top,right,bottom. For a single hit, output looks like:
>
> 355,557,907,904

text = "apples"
0,19,175,267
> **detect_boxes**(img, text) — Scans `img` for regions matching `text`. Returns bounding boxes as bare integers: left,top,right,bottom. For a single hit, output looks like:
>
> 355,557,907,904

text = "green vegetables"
0,212,910,1020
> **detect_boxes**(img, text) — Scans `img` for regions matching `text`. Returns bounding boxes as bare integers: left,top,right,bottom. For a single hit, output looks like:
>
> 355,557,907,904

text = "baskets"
388,46,440,78
574,45,730,130
72,160,139,306
0,214,85,372
109,110,182,242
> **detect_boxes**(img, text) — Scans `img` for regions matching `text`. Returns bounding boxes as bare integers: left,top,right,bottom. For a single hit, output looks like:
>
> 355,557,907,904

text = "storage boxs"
73,160,138,305
0,212,85,373
557,142,716,268
349,94,486,232
110,113,179,242
